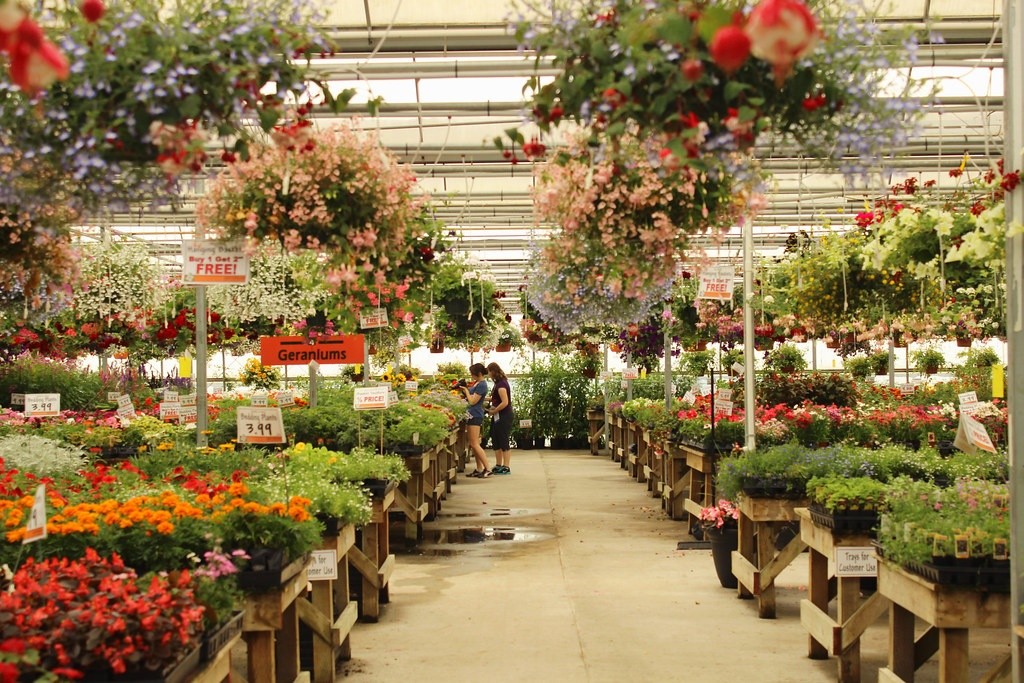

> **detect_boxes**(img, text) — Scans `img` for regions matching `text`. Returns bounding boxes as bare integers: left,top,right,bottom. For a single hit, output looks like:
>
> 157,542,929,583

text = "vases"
844,333,859,345
780,365,794,374
726,367,737,377
794,332,807,343
846,510,860,530
986,558,1010,585
704,530,738,590
957,337,972,347
954,559,980,584
827,340,840,349
894,334,908,348
758,340,775,351
496,340,512,352
683,341,708,352
430,340,445,353
861,510,875,529
832,509,846,529
583,368,596,379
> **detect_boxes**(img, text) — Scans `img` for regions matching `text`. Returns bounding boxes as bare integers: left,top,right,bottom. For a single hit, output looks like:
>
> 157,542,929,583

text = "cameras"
451,379,466,390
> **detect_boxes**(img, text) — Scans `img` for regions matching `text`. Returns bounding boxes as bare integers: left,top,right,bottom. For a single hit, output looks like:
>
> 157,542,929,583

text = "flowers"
0,362,414,429
0,0,1023,362
414,363,476,448
586,371,1011,573
0,429,413,683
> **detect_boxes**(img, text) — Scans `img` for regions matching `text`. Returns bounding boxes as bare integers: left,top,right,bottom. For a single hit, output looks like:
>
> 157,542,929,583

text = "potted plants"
867,350,899,375
908,345,946,379
339,365,370,383
842,357,871,380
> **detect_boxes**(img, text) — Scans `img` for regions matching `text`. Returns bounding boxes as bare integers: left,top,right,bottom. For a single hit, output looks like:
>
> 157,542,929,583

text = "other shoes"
497,466,510,473
492,464,501,473
478,469,493,478
466,469,480,477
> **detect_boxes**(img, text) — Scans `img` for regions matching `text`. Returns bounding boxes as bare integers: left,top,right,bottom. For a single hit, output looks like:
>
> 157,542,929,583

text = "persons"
483,362,514,476
458,363,494,478
